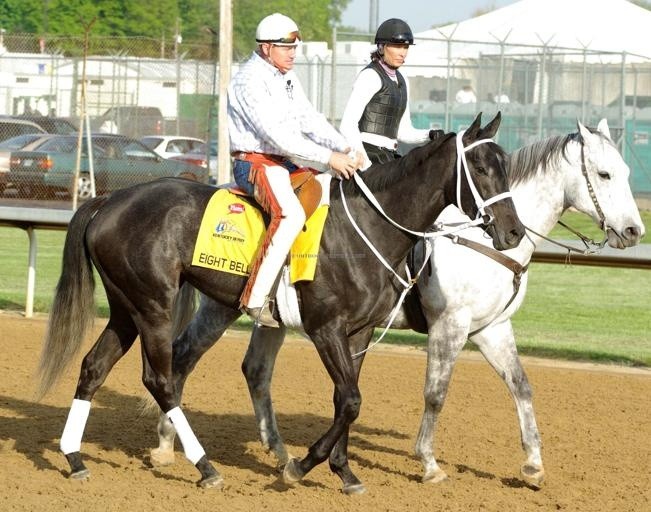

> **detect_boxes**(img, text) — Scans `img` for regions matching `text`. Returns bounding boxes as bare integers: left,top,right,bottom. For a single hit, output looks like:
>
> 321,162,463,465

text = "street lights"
437,24,604,138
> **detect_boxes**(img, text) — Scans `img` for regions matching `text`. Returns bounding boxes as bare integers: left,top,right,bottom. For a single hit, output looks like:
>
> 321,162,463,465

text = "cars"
0,106,218,200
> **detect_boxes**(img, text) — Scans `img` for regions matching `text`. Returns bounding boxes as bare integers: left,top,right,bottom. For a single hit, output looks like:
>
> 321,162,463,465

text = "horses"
137,117,645,489
33,111,526,496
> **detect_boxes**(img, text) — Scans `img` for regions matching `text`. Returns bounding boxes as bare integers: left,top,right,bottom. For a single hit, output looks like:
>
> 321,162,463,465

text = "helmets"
255,13,303,47
375,18,416,46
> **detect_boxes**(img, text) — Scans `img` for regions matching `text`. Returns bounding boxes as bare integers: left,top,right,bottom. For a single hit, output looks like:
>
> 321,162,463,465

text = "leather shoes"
239,304,280,330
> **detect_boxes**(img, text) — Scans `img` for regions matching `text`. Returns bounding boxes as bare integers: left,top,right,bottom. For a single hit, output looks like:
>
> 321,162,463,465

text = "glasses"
281,29,303,44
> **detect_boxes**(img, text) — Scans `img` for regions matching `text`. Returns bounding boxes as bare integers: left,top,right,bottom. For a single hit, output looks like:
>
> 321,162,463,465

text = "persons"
339,18,445,174
98,109,118,135
22,107,57,118
225,11,366,330
455,84,477,104
493,89,510,104
